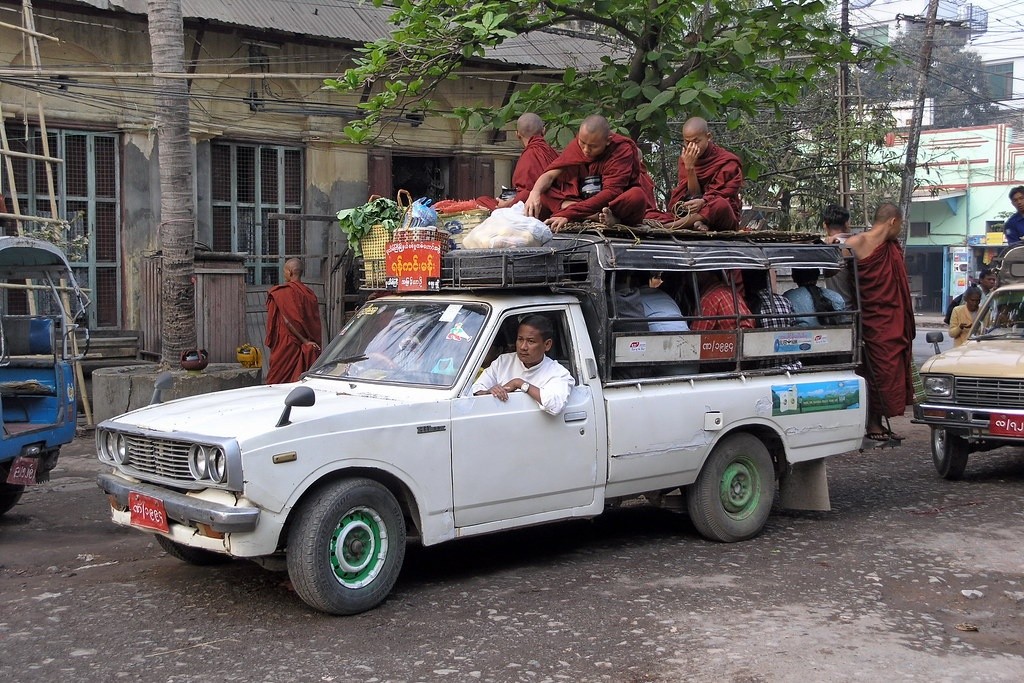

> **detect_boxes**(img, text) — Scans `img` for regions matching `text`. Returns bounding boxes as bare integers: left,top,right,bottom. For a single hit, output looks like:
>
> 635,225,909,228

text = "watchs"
520,381,530,392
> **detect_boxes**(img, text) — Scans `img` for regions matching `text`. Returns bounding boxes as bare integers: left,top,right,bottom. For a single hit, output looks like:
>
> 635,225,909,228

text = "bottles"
581,176,603,200
499,188,517,201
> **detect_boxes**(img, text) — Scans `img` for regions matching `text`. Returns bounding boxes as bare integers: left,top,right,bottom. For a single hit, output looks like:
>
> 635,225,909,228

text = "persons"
823,205,916,439
392,313,575,416
476,111,855,381
264,258,322,385
943,186,1024,348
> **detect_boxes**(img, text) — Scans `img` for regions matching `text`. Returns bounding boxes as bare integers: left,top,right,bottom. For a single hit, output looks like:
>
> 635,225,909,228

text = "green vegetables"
335,197,406,257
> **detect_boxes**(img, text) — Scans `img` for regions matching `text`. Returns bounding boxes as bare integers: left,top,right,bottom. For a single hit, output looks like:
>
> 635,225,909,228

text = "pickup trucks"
96,293,869,617
908,282,1023,479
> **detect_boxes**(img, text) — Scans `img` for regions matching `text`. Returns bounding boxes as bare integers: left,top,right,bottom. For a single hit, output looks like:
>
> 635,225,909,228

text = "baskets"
360,225,391,285
394,228,448,256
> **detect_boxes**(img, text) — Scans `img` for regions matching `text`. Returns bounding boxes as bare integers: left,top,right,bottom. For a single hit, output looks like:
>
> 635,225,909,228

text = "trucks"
0,233,79,515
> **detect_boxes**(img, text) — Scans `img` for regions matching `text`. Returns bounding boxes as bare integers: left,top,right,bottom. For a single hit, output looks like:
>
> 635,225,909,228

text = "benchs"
0,317,56,370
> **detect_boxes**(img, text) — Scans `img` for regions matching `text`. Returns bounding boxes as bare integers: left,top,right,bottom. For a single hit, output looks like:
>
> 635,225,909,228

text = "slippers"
884,428,906,439
865,432,888,441
